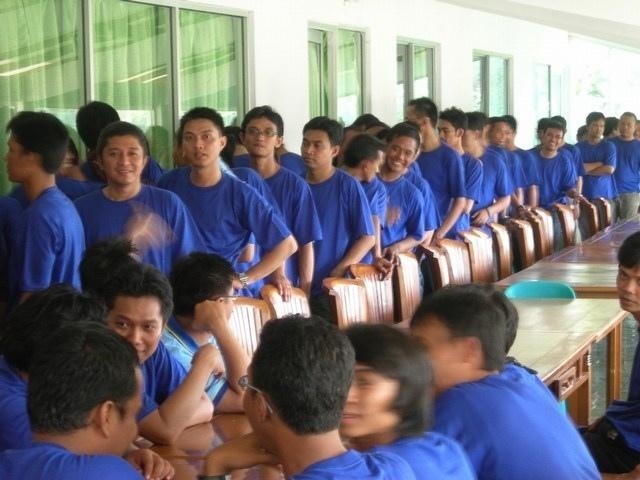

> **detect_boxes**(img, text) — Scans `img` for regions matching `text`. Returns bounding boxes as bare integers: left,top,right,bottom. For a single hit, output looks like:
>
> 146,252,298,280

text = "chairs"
207,196,614,384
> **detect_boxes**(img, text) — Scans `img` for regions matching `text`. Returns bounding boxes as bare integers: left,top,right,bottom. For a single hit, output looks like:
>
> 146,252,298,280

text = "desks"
392,213,640,426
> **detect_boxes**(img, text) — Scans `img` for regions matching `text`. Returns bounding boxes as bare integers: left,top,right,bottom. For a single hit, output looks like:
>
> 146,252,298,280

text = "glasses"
215,292,240,303
244,126,279,138
238,375,274,415
